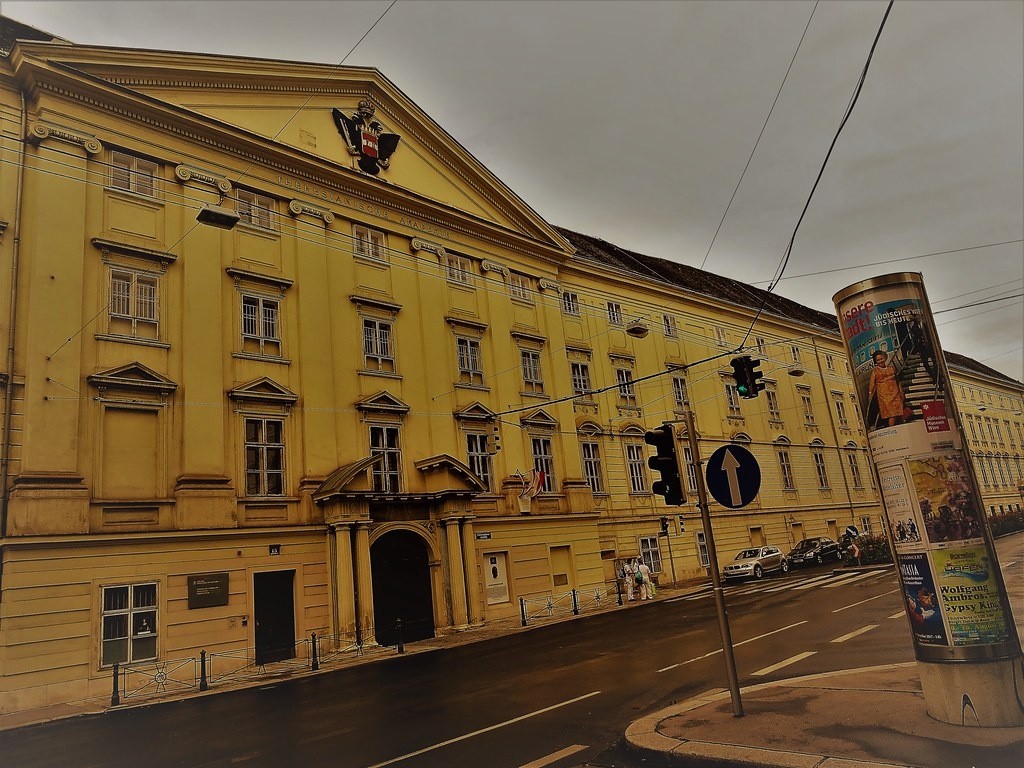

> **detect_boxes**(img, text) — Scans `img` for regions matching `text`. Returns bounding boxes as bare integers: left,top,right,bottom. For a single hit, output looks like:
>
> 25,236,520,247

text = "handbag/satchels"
900,401,914,421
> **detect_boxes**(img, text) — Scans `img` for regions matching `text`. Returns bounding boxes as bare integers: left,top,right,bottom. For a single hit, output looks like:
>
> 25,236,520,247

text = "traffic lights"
729,355,765,400
662,516,669,536
643,424,688,506
674,514,685,537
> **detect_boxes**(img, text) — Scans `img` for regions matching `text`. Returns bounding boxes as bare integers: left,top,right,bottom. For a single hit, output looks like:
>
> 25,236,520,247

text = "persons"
636,557,655,600
623,557,637,602
885,308,940,385
890,517,920,542
867,349,907,426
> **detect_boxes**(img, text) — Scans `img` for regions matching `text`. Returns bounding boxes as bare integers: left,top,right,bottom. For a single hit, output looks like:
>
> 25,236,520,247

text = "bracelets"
869,391,873,394
900,391,905,394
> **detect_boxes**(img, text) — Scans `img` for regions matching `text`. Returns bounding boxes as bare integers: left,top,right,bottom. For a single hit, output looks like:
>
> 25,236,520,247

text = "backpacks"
619,566,626,579
634,564,643,583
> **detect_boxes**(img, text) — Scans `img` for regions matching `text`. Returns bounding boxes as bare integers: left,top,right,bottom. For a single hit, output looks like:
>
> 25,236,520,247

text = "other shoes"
632,598,637,602
641,598,646,601
628,600,633,604
649,596,653,599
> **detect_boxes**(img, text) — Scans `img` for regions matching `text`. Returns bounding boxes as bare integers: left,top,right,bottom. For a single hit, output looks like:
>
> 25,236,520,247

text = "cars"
785,536,843,568
722,545,789,580
838,533,869,553
